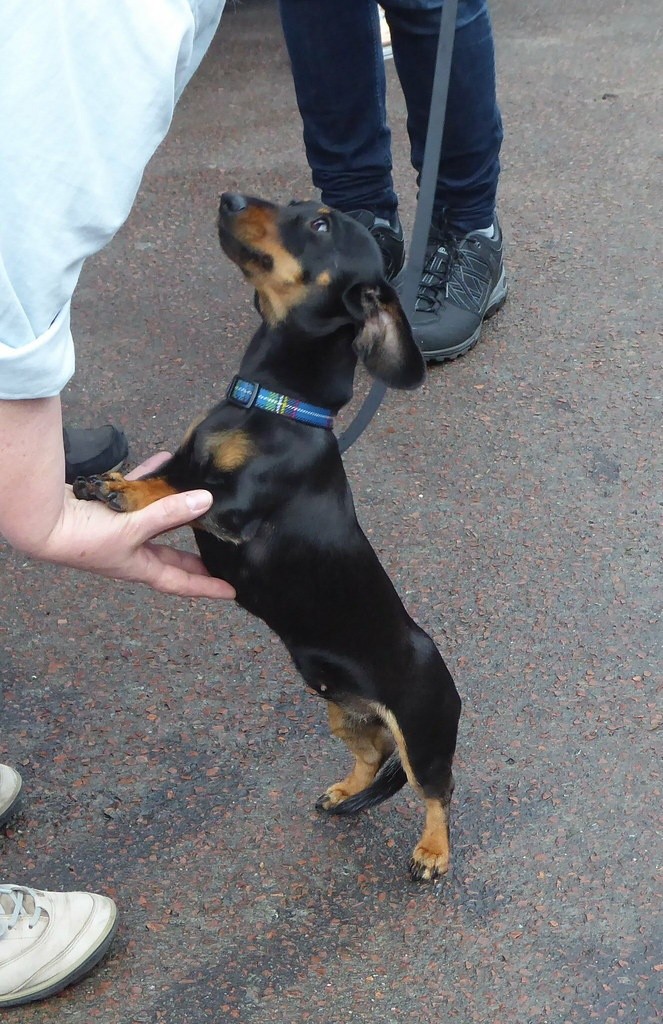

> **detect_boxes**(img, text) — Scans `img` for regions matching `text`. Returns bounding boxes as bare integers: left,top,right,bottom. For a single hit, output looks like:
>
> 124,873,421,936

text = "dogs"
68,189,466,882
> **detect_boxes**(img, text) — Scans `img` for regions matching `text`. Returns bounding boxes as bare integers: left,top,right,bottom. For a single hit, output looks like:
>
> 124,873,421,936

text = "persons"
279,0,510,364
0,0,236,1007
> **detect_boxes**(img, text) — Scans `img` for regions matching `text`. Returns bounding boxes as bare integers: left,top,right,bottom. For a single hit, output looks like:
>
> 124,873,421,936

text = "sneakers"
411,206,508,362
0,884,119,1007
343,208,405,283
63,425,128,484
0,764,22,818
